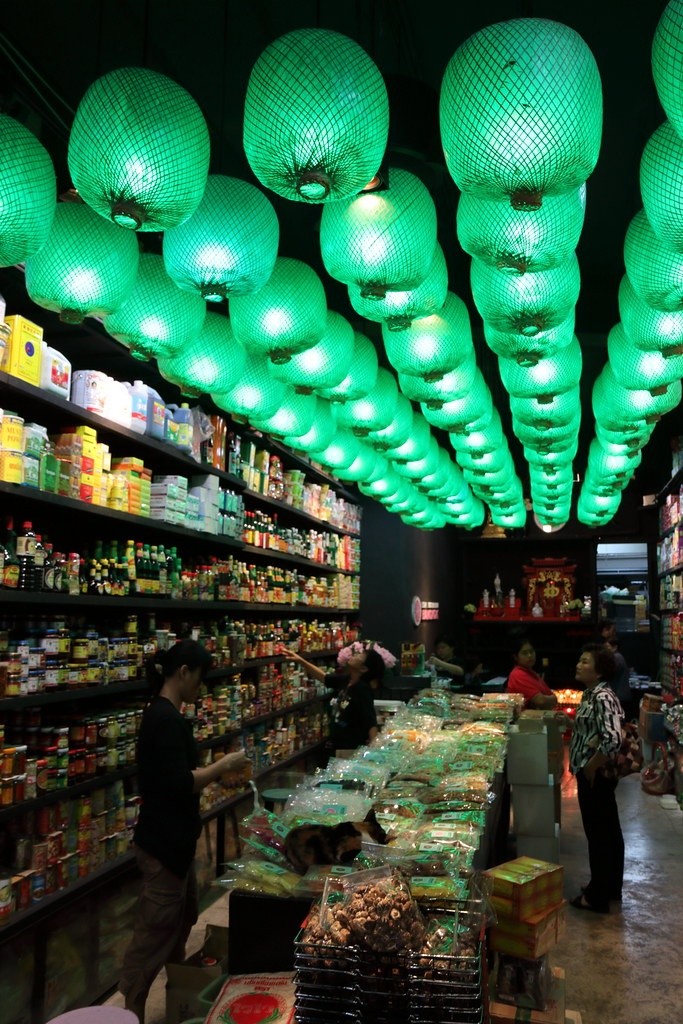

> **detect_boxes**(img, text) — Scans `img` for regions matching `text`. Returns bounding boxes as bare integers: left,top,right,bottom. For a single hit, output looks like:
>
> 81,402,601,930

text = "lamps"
0,0,683,529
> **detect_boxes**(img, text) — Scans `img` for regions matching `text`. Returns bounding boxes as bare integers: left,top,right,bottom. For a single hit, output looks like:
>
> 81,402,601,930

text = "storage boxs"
166,926,229,1023
480,856,582,1024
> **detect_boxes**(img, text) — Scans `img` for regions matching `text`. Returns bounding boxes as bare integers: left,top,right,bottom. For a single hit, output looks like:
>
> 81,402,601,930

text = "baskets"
291,898,485,1024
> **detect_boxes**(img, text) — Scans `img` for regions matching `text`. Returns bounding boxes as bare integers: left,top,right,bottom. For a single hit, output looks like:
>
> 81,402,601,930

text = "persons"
118,636,247,1024
506,637,560,709
280,646,385,759
569,643,628,913
596,623,616,643
605,635,634,722
428,634,471,687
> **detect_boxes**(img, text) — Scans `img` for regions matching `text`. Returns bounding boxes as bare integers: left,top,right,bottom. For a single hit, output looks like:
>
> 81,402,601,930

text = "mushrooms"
298,876,478,992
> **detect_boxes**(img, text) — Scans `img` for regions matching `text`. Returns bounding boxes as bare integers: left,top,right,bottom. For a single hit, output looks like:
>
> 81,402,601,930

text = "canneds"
0,363,360,914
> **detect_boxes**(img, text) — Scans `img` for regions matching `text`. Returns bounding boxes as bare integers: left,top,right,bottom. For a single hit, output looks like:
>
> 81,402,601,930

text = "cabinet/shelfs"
651,467,683,809
0,266,358,1023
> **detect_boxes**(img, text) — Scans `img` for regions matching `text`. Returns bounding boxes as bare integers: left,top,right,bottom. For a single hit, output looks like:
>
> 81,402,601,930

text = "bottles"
1,297,363,699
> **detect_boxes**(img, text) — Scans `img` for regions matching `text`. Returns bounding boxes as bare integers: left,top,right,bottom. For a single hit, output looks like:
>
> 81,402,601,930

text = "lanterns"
346,241,451,332
103,253,207,363
23,200,141,325
639,120,683,256
163,173,280,304
0,112,59,269
441,16,604,212
241,29,390,206
158,314,682,532
617,274,683,360
469,249,580,337
225,256,328,366
455,179,590,278
625,207,683,314
66,65,208,232
646,0,683,142
319,168,438,302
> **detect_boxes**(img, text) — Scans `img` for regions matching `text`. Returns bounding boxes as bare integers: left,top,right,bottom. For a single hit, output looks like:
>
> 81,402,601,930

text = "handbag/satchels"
599,721,643,778
642,742,671,793
